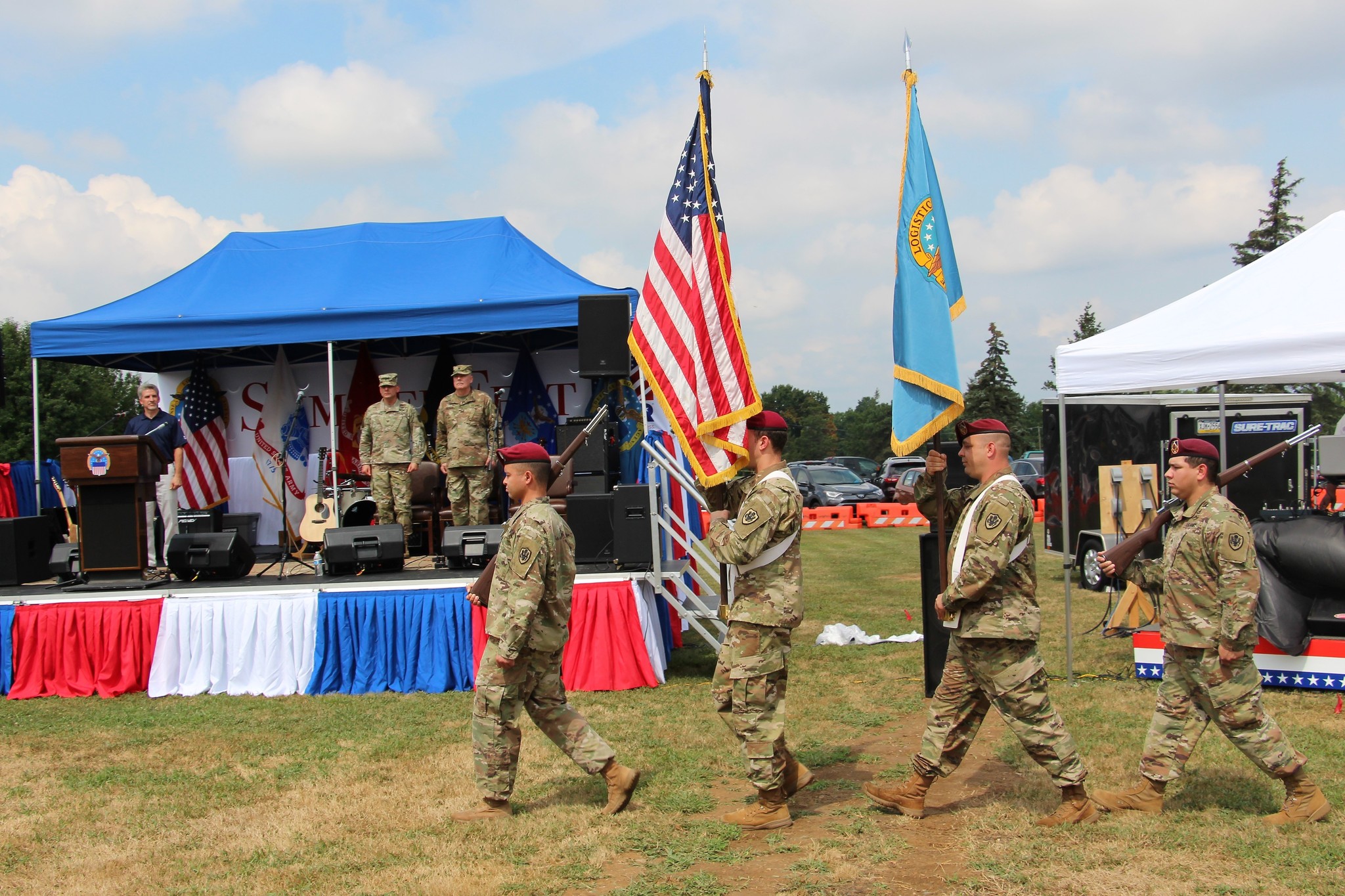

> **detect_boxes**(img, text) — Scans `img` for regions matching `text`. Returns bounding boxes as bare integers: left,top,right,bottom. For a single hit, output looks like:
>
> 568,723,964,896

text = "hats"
378,373,397,387
955,418,1010,446
494,442,551,466
1167,437,1219,462
747,410,788,432
451,364,472,377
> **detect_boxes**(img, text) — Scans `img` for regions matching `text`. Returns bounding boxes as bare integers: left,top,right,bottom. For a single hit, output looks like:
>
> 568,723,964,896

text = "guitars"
51,476,79,543
299,447,337,542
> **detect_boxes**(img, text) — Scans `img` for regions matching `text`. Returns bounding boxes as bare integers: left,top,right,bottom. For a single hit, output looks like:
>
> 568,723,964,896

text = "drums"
340,487,372,516
340,499,378,527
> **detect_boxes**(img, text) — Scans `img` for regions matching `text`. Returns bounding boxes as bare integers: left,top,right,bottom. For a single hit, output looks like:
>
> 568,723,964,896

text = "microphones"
296,391,304,403
494,388,505,395
145,422,168,435
114,411,130,418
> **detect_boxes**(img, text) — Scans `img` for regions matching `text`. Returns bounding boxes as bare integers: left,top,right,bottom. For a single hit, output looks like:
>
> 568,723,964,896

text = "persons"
450,441,641,820
123,384,188,577
707,410,816,831
862,418,1100,828
435,365,504,525
358,372,428,558
1090,437,1331,828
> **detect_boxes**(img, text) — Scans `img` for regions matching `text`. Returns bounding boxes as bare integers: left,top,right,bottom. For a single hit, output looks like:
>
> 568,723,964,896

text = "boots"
1258,767,1331,830
780,746,814,800
597,760,641,816
1031,783,1101,829
720,790,793,831
1090,775,1168,814
862,771,938,820
450,798,513,824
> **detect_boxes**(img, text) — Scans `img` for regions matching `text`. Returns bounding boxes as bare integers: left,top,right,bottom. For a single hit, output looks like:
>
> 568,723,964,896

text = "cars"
1006,450,1045,500
785,457,885,509
891,467,926,505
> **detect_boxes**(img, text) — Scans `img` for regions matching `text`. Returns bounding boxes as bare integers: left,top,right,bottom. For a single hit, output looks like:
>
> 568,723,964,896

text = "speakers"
554,422,661,564
579,293,630,381
0,515,48,587
441,525,506,570
407,523,428,557
323,523,404,576
160,509,256,580
0,350,6,408
48,542,81,582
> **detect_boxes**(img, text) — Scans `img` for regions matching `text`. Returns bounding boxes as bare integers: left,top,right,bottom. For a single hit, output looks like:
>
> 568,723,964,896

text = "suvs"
877,456,926,502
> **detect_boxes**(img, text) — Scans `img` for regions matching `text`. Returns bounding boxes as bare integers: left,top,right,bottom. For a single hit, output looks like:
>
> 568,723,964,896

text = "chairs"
372,460,502,557
507,453,575,524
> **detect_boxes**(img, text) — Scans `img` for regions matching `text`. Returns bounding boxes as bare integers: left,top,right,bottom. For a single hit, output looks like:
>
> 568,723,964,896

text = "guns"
1102,423,1323,578
469,404,608,609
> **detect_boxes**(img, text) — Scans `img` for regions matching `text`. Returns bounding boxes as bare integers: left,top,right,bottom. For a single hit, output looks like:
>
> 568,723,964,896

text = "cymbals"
337,473,371,481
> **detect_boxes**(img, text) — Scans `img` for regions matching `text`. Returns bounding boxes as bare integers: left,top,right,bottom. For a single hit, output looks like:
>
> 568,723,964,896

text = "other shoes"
149,566,157,570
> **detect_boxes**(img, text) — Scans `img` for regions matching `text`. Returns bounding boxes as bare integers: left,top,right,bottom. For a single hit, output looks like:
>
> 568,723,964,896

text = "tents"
1055,210,1345,684
29,216,650,515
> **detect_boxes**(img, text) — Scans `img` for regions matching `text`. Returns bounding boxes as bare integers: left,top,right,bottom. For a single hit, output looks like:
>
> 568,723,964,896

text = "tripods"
43,416,117,590
256,401,315,581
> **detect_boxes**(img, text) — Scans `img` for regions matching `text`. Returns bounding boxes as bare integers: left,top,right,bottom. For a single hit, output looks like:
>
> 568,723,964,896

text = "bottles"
313,552,323,576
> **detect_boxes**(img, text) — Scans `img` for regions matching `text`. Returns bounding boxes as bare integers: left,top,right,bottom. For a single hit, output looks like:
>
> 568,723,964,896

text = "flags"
626,72,762,487
339,344,383,526
501,333,558,455
177,354,230,509
253,344,310,542
421,335,458,489
889,72,971,457
